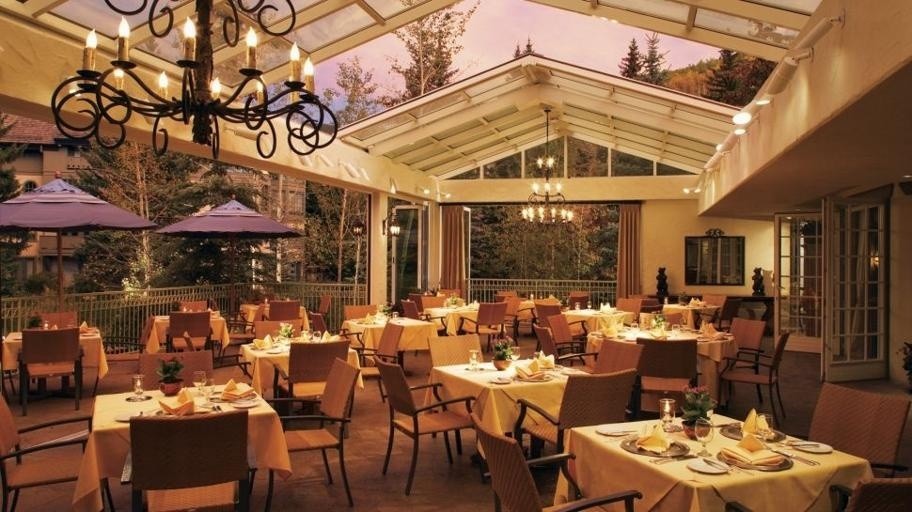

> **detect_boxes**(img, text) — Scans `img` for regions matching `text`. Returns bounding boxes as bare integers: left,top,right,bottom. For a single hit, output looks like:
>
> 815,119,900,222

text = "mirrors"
684,228,745,285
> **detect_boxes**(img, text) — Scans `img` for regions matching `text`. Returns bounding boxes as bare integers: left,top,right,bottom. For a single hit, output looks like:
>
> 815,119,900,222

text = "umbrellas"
1,170,159,328
155,193,307,316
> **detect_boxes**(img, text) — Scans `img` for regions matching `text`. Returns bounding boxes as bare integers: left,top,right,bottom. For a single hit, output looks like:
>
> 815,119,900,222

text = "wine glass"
313,331,321,344
132,375,145,401
392,312,399,322
511,347,520,368
631,323,638,330
695,419,713,457
193,371,207,392
671,324,680,340
587,301,592,311
659,398,675,430
754,413,775,453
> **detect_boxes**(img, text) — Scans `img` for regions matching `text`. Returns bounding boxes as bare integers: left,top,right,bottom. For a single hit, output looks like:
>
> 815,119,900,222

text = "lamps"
683,9,845,194
51,0,338,157
382,212,401,237
521,109,574,223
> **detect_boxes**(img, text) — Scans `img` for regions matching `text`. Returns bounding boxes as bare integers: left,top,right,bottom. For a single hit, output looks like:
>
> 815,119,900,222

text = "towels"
741,408,774,437
722,435,785,466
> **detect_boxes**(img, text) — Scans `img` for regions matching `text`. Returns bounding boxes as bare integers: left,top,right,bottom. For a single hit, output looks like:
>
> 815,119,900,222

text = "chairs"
721,331,792,427
725,478,912,511
792,383,912,491
728,317,766,403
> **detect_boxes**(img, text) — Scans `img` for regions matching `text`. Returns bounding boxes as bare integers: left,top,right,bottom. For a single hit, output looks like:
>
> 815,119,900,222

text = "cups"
469,350,479,372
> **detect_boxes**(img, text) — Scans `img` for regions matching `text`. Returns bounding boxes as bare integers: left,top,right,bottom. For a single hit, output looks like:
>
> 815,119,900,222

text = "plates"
154,316,169,319
596,422,644,436
491,378,510,384
720,424,785,443
717,452,793,471
151,406,224,416
688,458,729,474
514,373,552,382
231,400,259,409
268,349,281,354
207,392,256,403
794,441,833,454
621,439,690,457
114,414,149,422
